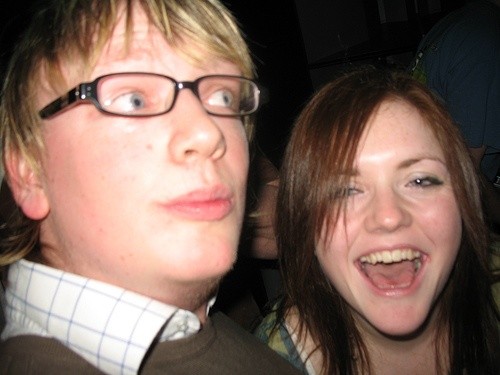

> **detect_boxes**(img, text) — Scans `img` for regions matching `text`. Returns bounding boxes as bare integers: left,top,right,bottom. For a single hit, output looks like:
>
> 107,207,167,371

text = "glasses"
38,71,263,118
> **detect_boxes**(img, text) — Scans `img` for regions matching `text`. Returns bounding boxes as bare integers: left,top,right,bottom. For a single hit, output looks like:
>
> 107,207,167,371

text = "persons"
408,0,500,235
215,113,285,333
0,0,301,375
252,68,500,375
